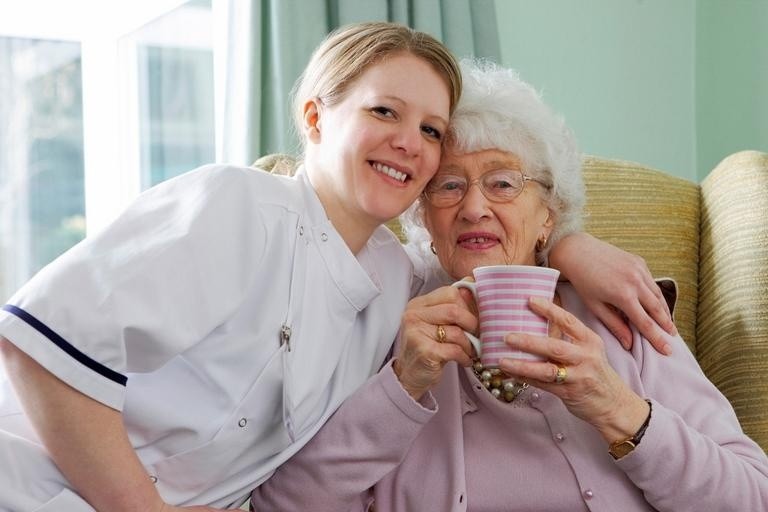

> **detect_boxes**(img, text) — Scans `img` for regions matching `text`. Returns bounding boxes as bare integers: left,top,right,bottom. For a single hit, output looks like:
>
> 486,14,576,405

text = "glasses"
418,167,552,209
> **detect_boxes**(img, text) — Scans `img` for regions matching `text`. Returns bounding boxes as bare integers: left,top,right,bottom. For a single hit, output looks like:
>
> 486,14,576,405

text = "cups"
446,265,562,372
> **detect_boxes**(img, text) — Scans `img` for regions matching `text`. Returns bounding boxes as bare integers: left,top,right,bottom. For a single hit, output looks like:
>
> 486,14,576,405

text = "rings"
555,367,567,382
436,325,447,345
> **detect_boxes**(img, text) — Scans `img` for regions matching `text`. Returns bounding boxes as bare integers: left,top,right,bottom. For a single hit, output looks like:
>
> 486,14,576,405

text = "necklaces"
471,359,530,404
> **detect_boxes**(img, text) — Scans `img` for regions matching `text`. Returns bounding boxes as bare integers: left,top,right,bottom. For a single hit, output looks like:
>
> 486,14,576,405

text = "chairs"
247,141,768,459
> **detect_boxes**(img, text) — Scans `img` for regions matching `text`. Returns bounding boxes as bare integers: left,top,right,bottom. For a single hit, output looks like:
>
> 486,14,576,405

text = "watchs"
609,399,652,461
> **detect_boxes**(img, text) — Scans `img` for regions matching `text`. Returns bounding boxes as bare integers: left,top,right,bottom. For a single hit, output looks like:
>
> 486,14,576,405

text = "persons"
0,22,676,512
250,60,768,511
